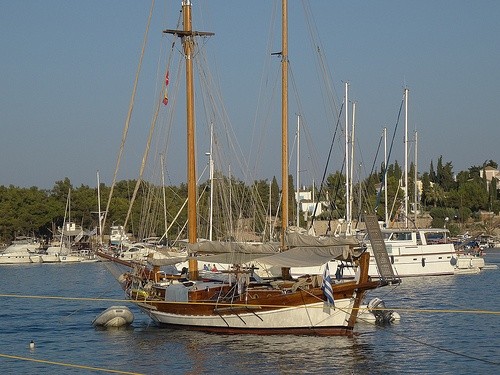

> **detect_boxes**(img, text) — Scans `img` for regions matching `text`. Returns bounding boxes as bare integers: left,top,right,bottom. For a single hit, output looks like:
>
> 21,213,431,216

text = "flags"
321,263,335,311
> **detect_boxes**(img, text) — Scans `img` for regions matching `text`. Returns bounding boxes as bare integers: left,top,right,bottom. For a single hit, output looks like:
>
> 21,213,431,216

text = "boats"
290,0,480,280
197,268,222,275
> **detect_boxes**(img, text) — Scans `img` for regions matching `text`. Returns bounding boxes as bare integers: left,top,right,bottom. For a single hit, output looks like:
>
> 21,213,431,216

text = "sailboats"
91,0,401,335
0,188,91,264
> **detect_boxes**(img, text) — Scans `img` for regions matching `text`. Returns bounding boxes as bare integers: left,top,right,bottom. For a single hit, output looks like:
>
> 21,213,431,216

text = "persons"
210,263,218,272
204,264,208,270
207,264,212,270
